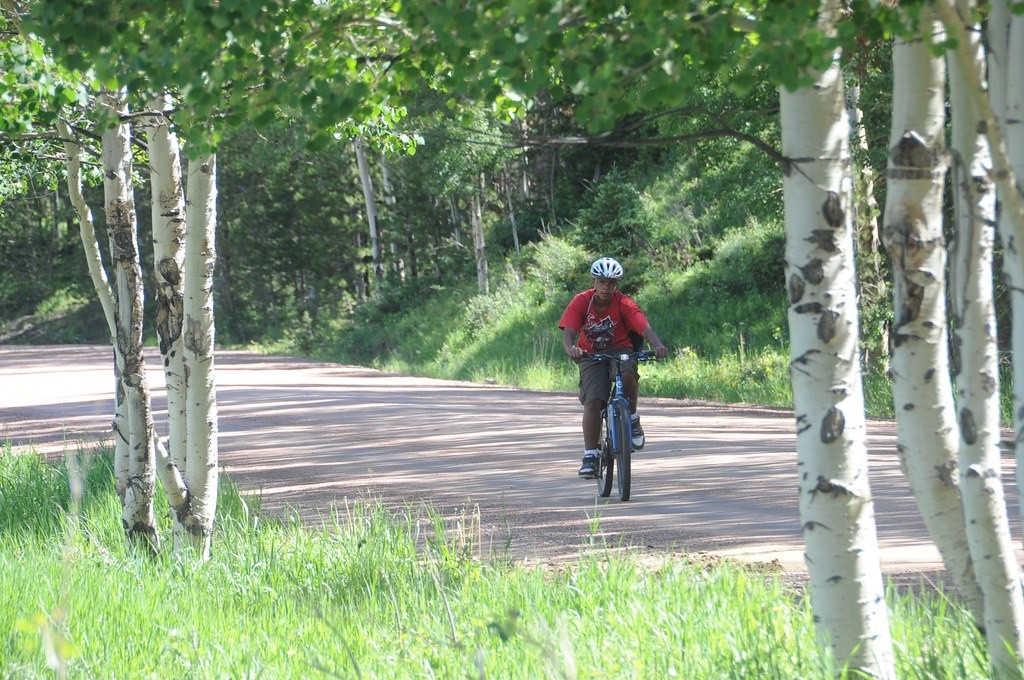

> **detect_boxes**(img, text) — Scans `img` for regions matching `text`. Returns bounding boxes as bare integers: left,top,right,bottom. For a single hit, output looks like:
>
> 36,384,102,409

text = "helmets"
590,257,623,280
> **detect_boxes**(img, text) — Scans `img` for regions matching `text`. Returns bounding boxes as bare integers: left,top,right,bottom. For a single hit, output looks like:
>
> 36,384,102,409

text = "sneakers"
630,411,645,449
578,448,605,479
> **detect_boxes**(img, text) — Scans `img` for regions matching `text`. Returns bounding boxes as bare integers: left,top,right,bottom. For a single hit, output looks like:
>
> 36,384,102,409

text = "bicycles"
572,350,660,502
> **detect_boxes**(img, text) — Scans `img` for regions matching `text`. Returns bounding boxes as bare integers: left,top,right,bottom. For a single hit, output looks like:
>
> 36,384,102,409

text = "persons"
557,257,669,478
